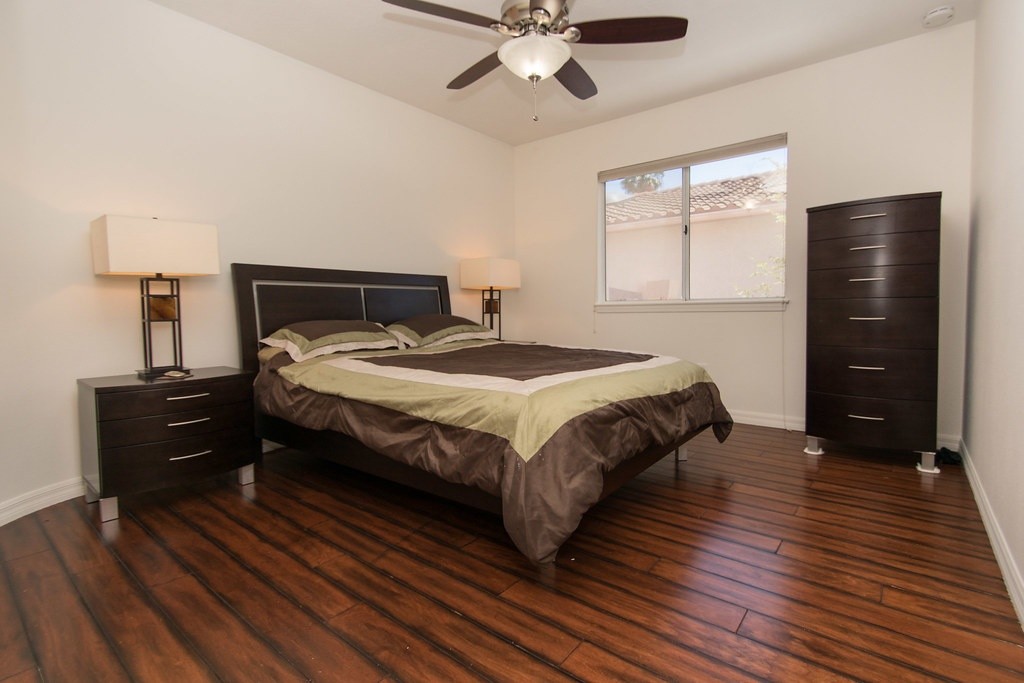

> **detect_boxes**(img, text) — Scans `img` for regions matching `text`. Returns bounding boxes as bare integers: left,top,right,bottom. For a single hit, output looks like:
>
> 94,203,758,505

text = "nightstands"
75,365,257,526
502,339,538,346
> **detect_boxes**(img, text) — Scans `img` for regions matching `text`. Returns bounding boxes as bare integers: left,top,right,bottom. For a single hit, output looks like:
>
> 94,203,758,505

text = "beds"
228,262,702,547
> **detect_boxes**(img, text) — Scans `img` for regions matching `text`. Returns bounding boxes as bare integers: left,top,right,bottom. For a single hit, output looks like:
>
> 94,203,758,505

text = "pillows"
257,320,405,363
386,314,496,349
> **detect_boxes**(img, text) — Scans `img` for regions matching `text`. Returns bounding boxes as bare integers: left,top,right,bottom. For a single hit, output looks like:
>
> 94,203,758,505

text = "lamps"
459,258,521,338
90,211,219,379
495,0,572,121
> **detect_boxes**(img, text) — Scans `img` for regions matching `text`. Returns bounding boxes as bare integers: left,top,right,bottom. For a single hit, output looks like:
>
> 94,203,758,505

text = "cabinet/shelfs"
805,191,943,471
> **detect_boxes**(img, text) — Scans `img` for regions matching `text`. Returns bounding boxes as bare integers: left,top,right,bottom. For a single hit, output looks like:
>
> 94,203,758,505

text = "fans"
382,0,688,101
